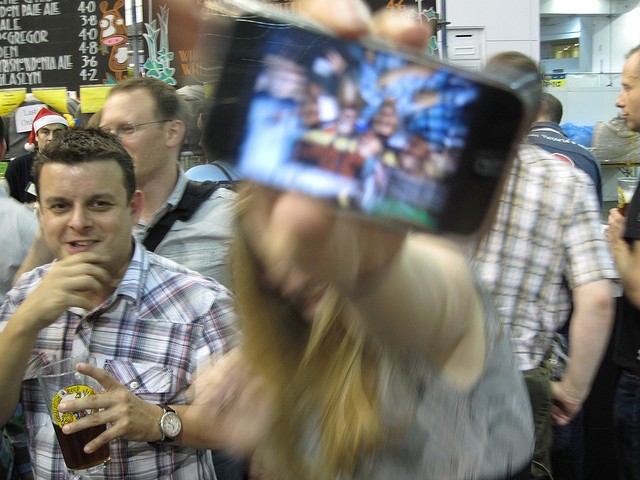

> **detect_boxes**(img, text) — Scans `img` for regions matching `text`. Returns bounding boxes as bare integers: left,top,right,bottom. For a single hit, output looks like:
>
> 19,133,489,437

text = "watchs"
148,403,184,447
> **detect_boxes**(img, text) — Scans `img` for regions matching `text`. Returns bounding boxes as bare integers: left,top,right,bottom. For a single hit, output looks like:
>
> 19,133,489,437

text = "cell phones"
198,10,527,239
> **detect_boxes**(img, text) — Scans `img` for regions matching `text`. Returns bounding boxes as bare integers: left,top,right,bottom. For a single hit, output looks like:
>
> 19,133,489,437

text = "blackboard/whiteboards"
0,0,124,92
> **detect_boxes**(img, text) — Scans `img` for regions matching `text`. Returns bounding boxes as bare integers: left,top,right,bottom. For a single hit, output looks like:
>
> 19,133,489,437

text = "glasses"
100,119,172,136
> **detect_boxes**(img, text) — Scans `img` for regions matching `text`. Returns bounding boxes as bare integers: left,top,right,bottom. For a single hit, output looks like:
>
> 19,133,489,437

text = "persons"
528,90,603,213
174,84,205,156
194,0,537,479
606,43,640,311
4,106,70,203
0,124,245,479
466,50,625,480
185,94,244,183
0,114,40,309
97,77,244,292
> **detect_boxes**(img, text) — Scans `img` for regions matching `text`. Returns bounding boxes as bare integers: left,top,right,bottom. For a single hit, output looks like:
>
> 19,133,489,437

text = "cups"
616,176,636,217
37,356,111,473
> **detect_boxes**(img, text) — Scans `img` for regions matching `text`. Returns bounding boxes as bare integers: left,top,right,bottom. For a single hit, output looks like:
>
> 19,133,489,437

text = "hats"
23,106,69,153
